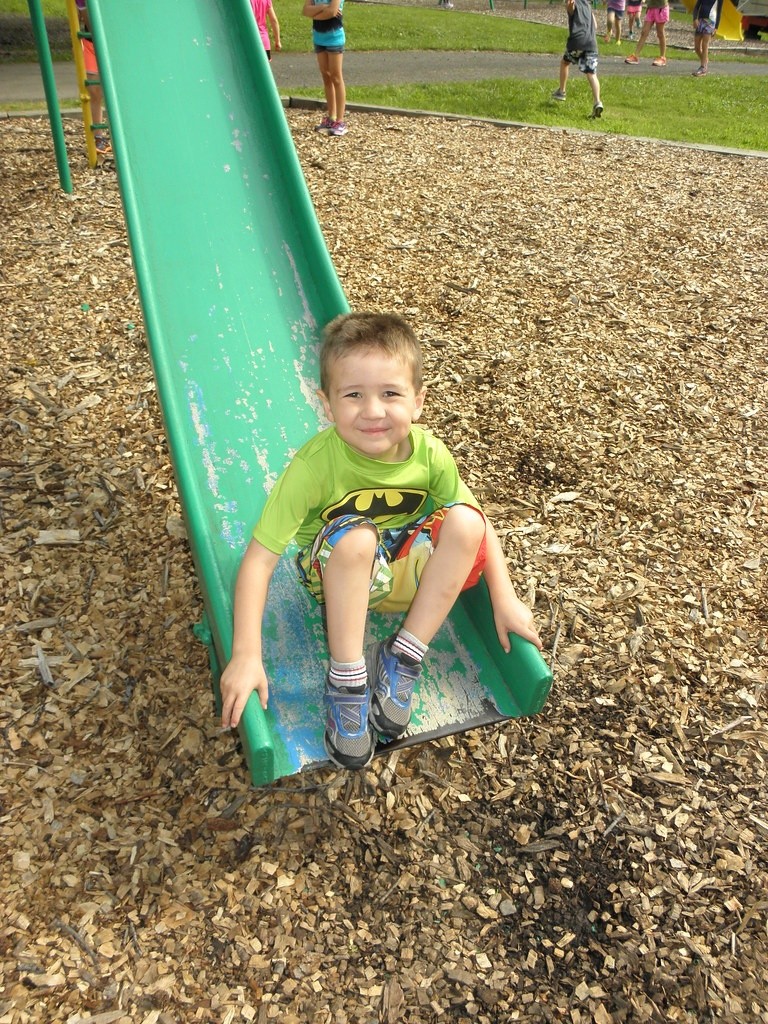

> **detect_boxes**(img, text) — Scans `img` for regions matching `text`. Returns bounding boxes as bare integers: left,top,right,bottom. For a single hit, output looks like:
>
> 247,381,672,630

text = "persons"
76,0,112,153
302,0,349,136
627,0,642,40
605,0,625,46
216,308,543,771
625,0,669,67
692,0,724,77
551,0,604,119
250,0,282,62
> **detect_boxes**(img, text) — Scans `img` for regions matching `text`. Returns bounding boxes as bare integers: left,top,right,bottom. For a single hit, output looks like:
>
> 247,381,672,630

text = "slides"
682,0,744,42
89,1,557,790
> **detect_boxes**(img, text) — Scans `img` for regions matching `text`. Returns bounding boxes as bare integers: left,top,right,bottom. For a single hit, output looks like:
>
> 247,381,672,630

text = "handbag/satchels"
695,17,716,34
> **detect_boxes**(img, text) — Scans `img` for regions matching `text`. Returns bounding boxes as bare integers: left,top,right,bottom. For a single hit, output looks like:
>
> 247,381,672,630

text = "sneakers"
691,65,707,77
590,102,604,120
368,633,423,740
551,88,567,101
625,55,639,65
328,120,348,135
314,117,336,137
322,665,378,770
652,56,667,66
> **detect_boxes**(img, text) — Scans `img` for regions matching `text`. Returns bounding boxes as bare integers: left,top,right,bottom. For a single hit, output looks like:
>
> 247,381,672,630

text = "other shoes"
615,40,621,46
604,36,611,43
94,137,109,151
637,21,642,28
627,31,634,40
444,3,454,10
